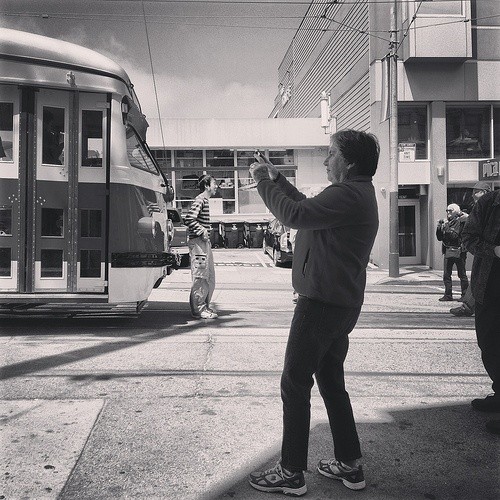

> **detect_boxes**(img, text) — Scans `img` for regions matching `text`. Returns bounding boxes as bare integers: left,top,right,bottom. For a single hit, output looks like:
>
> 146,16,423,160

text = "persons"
450,182,492,317
289,228,299,304
184,175,218,319
436,203,469,301
462,189,500,430
248,129,381,496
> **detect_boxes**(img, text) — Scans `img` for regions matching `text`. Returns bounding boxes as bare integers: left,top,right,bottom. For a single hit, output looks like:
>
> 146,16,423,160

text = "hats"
470,182,491,190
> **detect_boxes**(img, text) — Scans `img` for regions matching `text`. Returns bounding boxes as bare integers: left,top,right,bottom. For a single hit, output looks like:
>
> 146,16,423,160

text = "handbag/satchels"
445,245,461,259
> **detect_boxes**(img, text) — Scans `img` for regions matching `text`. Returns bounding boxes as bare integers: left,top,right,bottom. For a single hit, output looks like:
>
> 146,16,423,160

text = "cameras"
438,219,444,226
255,149,261,162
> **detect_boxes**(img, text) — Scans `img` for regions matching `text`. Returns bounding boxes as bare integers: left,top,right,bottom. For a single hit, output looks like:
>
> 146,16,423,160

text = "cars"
262,218,295,268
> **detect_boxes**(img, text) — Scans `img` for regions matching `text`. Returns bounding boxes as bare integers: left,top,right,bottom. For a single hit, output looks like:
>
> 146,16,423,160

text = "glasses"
446,209,452,212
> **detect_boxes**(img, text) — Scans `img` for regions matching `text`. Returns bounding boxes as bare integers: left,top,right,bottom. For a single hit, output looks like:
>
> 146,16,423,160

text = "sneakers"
191,308,223,319
318,458,366,490
247,458,308,497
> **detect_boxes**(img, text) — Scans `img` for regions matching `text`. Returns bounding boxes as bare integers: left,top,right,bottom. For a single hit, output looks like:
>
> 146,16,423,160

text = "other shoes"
439,294,454,301
450,306,473,317
485,419,499,434
470,392,500,412
458,296,465,302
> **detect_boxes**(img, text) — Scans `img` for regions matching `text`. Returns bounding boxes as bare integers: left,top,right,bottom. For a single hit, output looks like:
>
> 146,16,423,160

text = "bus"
0,26,190,306
